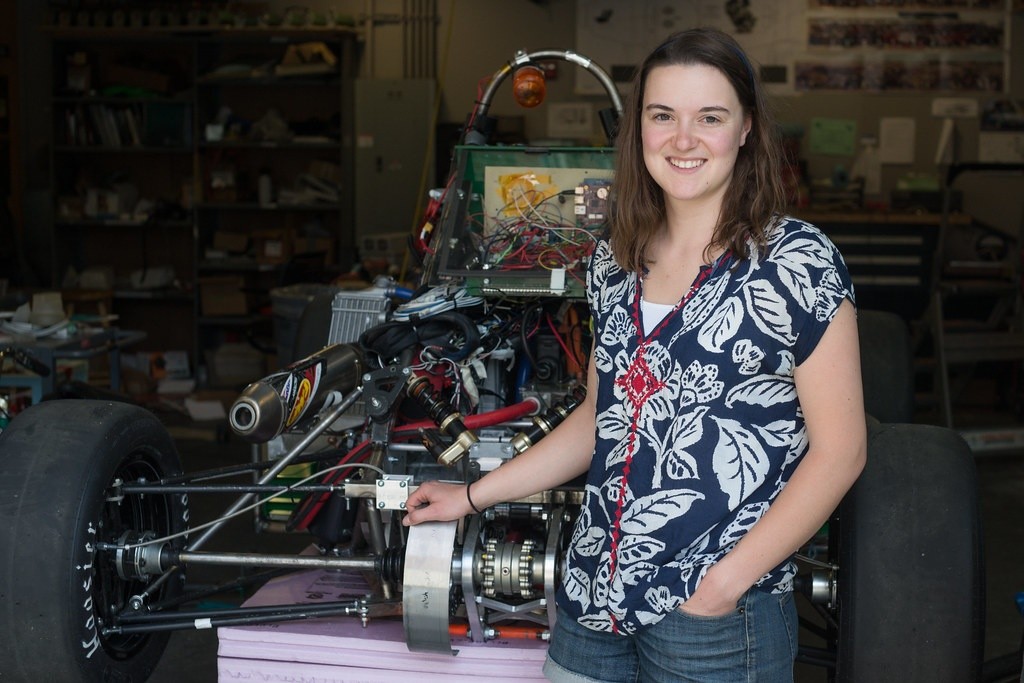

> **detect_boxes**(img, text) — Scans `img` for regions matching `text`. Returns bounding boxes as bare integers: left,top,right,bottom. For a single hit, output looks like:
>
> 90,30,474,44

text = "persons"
392,28,867,683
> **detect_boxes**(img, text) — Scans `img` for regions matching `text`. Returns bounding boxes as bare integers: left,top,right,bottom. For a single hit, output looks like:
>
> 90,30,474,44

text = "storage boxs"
203,277,246,316
214,230,248,251
81,265,114,289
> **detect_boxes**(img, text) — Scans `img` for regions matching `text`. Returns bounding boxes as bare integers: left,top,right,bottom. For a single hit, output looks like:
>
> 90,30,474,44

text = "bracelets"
467,481,481,515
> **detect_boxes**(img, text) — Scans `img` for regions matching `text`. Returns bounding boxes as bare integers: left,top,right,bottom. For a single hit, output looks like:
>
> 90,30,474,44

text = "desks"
785,208,971,318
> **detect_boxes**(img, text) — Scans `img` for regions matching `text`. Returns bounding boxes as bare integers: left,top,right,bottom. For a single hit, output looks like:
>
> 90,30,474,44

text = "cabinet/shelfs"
52,31,358,387
